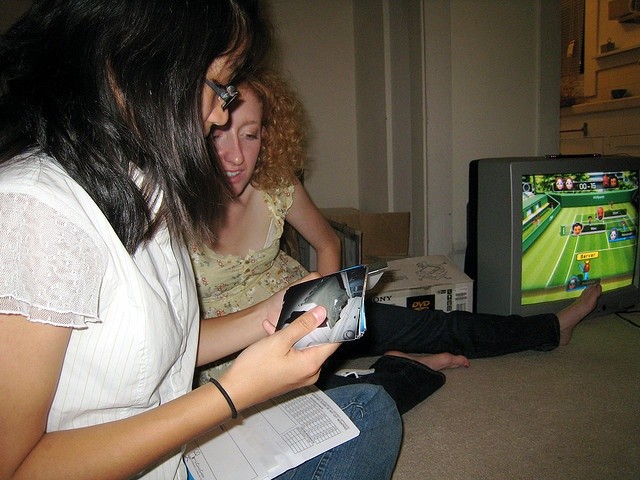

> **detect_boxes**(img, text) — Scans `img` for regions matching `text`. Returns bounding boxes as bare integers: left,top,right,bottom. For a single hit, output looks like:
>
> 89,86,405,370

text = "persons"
187,63,601,415
0,0,403,480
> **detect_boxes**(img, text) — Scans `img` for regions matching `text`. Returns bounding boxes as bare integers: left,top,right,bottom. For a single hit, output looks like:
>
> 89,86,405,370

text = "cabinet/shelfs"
559,96,640,157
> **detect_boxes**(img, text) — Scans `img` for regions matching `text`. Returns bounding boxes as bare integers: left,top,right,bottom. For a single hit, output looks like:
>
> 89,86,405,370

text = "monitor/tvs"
468,156,638,318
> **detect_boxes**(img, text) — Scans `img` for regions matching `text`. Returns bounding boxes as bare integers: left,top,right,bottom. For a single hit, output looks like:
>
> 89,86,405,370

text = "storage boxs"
282,207,410,265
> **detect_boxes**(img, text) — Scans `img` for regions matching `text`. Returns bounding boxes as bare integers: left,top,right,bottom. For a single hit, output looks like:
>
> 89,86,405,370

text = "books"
182,385,361,480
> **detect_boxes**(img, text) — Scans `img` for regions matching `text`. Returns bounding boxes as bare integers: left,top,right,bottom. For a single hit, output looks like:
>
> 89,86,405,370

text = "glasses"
204,79,239,111
